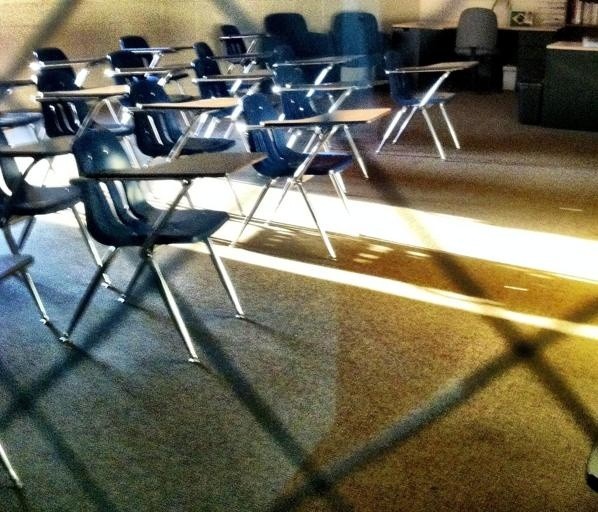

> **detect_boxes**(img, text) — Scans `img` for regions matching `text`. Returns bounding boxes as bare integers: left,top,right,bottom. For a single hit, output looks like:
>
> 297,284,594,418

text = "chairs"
1,12,382,241
229,95,363,260
59,128,246,364
375,50,461,162
1,216,35,305
453,7,499,93
0,118,127,317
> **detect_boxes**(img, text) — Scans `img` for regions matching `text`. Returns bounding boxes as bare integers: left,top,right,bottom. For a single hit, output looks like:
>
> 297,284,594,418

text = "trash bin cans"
517,78,544,126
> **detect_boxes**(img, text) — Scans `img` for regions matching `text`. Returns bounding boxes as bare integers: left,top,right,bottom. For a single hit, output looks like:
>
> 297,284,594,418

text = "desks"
2,134,82,160
259,107,391,125
391,22,598,132
383,61,480,74
85,152,270,180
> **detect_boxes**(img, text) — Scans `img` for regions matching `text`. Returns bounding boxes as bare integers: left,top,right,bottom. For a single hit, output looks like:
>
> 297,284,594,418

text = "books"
582,36,598,47
569,0,598,26
